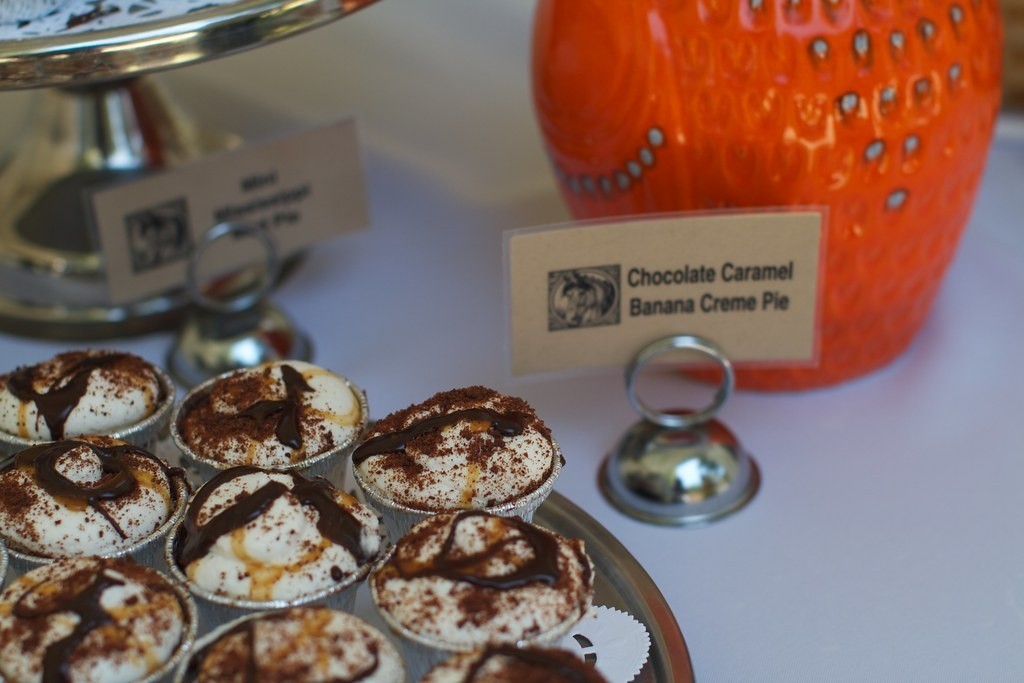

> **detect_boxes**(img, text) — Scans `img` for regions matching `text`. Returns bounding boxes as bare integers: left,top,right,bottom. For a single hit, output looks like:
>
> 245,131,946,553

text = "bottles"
533,1,1005,396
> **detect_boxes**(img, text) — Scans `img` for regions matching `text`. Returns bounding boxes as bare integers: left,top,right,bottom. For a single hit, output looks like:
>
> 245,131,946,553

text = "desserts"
0,350,612,683
